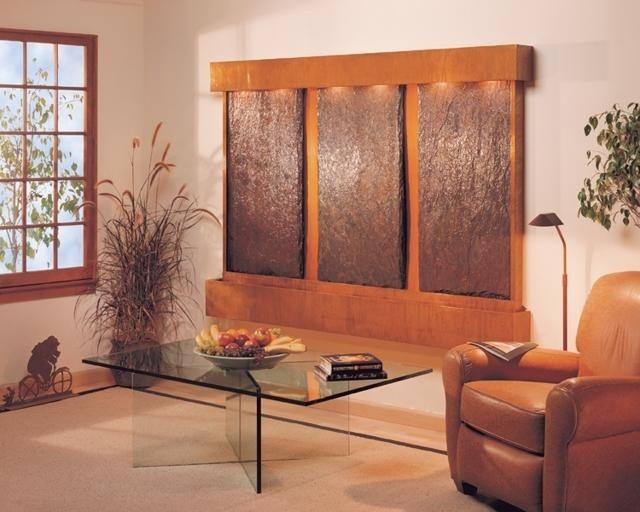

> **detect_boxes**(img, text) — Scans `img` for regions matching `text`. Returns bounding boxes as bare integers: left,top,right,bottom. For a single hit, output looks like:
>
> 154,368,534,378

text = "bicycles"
17,357,72,404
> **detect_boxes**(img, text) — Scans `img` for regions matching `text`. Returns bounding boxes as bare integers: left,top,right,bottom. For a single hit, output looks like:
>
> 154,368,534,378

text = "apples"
218,327,278,356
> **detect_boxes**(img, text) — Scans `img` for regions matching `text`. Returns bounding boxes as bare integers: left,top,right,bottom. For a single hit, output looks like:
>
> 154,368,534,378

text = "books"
312,352,389,381
467,340,541,362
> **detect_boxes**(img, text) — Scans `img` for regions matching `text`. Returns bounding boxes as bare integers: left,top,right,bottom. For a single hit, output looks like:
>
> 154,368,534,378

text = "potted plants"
70,122,222,389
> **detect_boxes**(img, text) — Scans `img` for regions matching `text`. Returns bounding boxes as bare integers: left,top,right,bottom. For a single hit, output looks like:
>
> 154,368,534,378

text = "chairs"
442,269,640,512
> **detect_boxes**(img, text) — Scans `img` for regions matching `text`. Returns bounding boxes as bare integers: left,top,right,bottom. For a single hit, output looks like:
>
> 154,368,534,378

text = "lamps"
528,212,570,350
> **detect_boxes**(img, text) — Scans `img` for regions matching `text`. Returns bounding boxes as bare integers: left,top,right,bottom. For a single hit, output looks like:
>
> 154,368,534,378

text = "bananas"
263,336,307,354
193,323,220,351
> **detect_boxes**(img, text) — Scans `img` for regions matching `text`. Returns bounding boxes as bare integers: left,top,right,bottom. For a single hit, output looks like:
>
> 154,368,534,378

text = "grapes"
251,347,269,368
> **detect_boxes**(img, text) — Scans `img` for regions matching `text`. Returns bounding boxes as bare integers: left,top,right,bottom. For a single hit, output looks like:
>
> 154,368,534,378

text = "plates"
194,346,288,371
